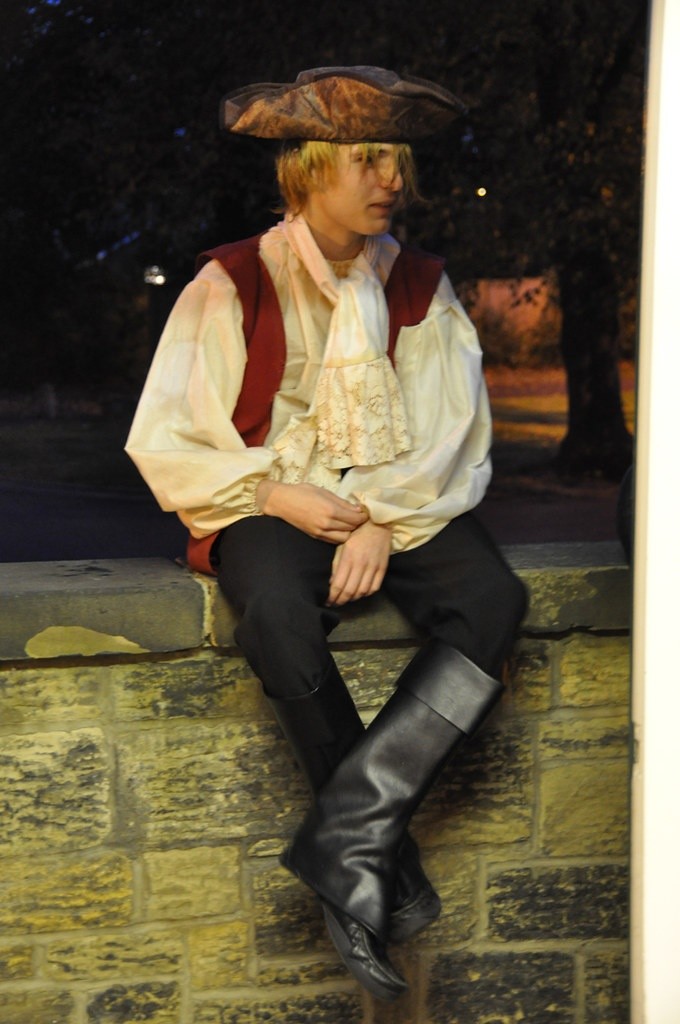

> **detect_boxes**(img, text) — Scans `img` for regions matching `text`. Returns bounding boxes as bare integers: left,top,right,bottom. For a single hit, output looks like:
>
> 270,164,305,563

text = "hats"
222,63,468,145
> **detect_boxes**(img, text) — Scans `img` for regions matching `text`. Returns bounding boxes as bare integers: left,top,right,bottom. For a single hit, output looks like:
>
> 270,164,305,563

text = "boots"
279,640,502,1000
263,655,443,941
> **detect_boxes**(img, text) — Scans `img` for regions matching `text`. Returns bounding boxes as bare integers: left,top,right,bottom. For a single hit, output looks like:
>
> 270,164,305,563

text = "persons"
123,65,531,1002
